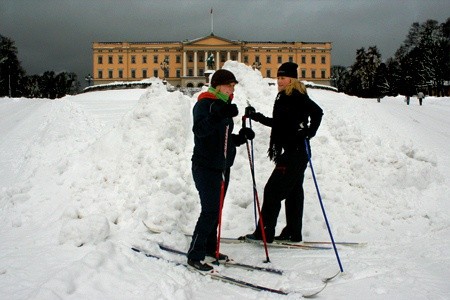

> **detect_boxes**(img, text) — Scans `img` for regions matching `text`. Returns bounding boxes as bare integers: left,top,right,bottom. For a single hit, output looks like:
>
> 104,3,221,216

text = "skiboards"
185,225,363,250
131,236,342,298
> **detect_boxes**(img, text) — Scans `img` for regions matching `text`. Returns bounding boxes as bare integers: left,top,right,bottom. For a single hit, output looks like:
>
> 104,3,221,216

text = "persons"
245,62,324,244
406,89,410,105
418,91,424,105
186,69,255,274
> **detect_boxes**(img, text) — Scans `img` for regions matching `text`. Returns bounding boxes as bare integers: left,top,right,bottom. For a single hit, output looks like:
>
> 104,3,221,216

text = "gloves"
245,107,260,122
212,103,238,120
236,127,255,146
298,127,314,139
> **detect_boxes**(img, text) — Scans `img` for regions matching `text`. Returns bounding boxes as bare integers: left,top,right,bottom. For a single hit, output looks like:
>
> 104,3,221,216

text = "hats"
277,62,298,79
211,69,238,89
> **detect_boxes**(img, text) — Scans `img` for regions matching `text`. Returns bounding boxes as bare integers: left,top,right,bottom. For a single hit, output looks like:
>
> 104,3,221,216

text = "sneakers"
205,252,229,261
274,229,302,242
186,258,214,272
245,231,274,244
